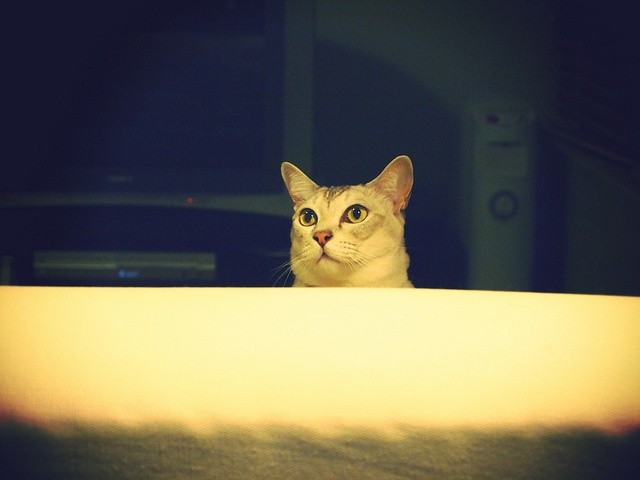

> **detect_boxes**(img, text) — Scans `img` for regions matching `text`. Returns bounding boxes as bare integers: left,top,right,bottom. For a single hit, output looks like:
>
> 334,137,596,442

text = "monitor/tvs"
1,0,319,220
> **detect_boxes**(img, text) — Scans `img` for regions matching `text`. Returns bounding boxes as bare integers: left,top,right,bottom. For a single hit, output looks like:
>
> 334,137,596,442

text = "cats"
269,155,415,288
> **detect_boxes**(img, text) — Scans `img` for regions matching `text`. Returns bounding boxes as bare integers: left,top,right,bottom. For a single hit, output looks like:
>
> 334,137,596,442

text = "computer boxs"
459,94,540,291
